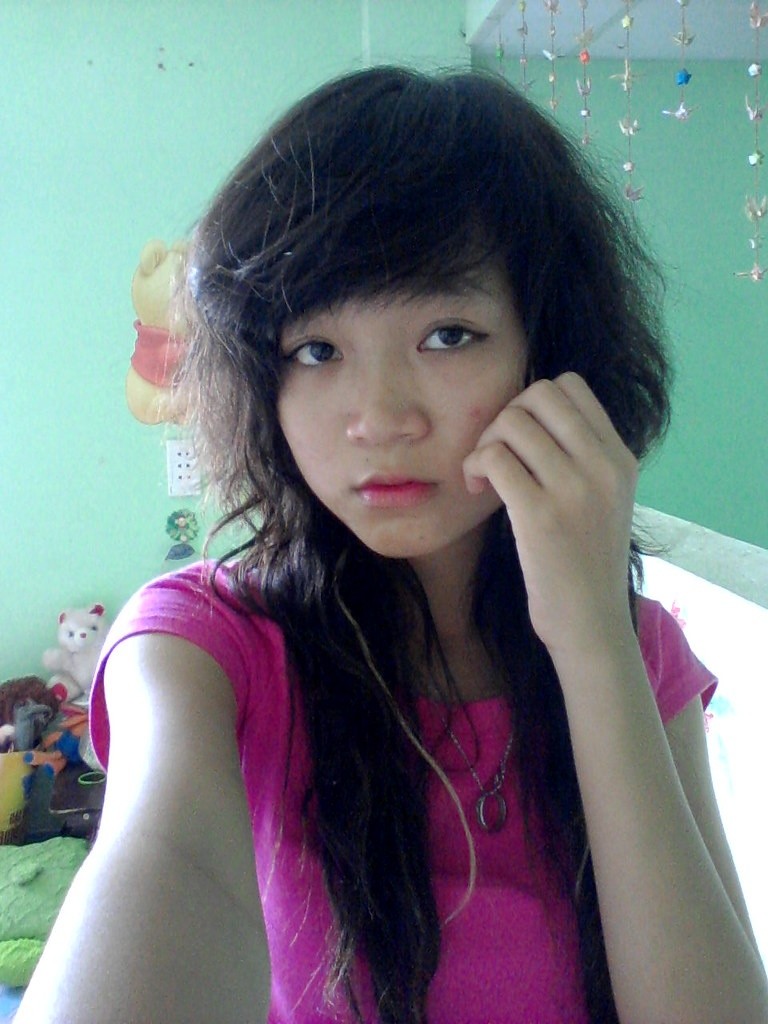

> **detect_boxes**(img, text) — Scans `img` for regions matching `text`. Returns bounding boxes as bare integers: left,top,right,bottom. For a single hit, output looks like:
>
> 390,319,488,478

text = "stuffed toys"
0,602,111,753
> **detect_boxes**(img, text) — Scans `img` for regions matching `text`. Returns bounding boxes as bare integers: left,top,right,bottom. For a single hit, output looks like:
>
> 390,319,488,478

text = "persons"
9,62,768,1022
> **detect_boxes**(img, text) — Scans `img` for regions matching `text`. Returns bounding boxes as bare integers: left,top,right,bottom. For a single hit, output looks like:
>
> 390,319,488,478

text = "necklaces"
417,667,517,833
